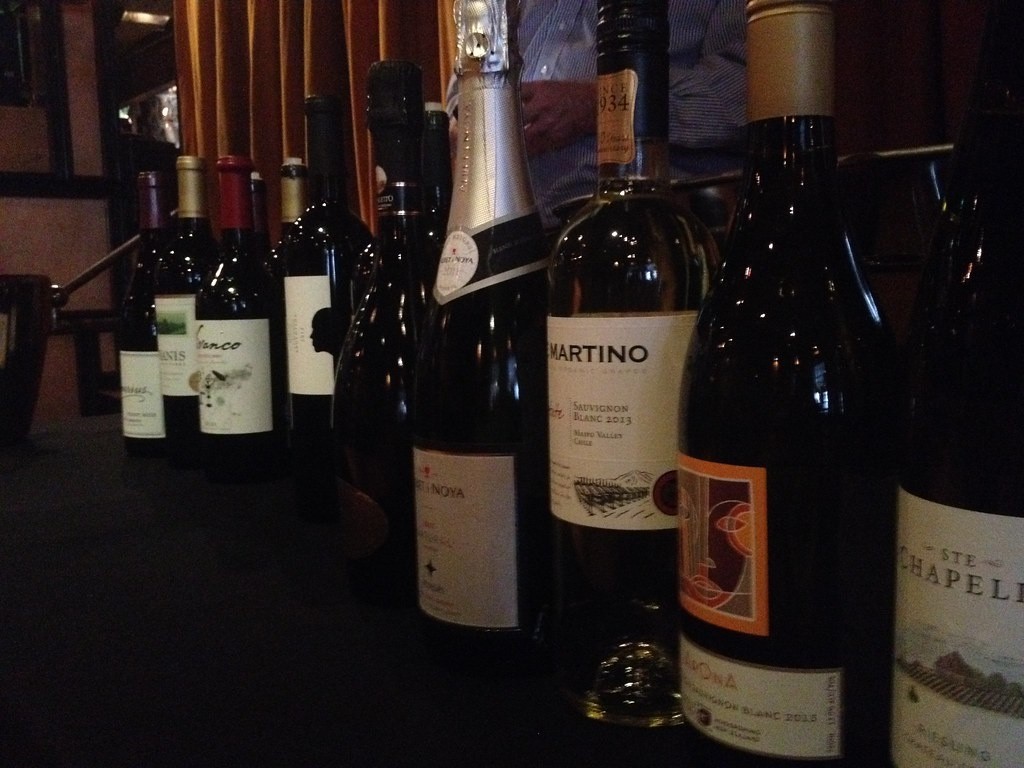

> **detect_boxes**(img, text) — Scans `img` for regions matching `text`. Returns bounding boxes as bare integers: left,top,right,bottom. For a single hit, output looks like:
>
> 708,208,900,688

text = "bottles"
891,0,1024,768
120,60,448,469
677,0,890,767
414,0,558,664
544,0,692,732
